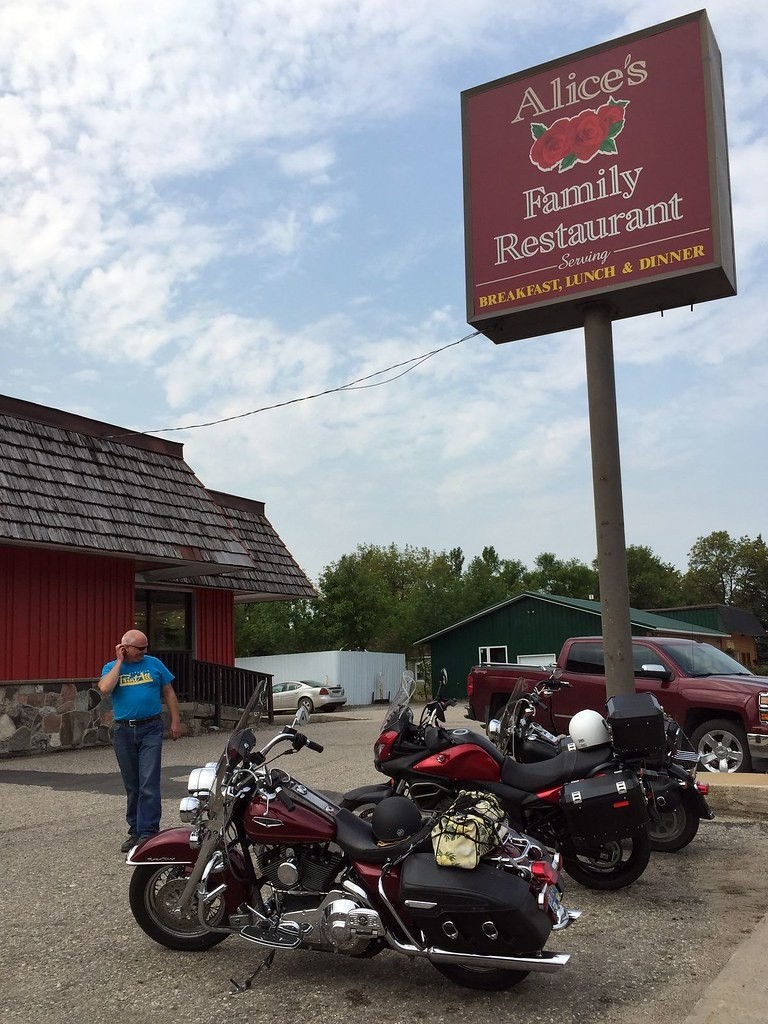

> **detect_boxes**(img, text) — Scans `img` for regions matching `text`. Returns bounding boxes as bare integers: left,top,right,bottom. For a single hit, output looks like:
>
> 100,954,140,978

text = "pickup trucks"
465,635,768,774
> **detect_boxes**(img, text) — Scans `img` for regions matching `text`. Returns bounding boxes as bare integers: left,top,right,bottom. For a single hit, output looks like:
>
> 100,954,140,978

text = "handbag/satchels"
431,790,507,870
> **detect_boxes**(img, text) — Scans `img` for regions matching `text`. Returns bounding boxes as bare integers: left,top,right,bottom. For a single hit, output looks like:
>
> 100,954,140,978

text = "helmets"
569,710,611,751
372,797,421,842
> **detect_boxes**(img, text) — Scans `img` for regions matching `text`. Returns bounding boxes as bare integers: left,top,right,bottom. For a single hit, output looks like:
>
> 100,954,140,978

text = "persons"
98,629,180,852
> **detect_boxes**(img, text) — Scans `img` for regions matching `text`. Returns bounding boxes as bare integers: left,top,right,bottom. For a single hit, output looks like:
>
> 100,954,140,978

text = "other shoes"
121,836,138,852
137,837,148,845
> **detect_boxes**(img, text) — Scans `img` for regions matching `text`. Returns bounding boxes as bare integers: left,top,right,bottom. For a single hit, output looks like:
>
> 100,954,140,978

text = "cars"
270,679,347,715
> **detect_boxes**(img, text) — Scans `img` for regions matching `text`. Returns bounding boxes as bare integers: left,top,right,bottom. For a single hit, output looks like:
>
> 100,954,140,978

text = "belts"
116,715,161,727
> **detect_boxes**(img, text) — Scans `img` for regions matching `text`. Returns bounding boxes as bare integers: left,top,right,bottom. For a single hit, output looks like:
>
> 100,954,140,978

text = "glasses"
126,645,148,651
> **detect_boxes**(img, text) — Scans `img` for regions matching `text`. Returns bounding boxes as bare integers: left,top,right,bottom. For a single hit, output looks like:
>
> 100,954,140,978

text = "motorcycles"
338,667,670,893
126,707,581,989
482,662,715,853
